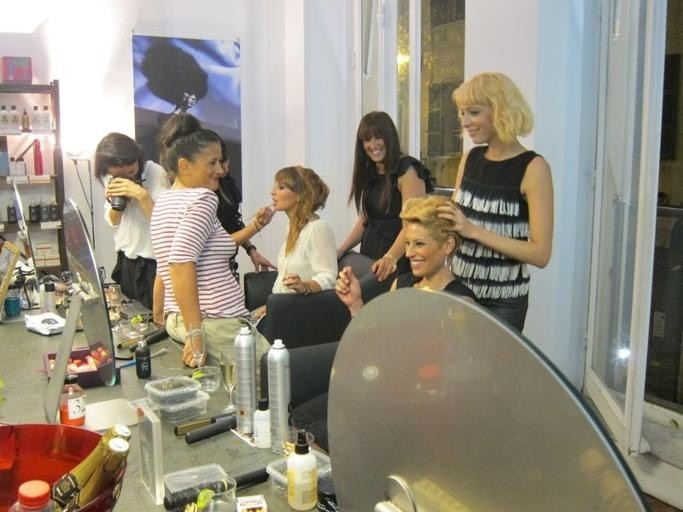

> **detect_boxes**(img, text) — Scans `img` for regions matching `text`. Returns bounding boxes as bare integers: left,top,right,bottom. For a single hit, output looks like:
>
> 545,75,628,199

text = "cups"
195,476,238,512
190,365,221,392
110,177,127,210
7,202,59,224
39,290,56,313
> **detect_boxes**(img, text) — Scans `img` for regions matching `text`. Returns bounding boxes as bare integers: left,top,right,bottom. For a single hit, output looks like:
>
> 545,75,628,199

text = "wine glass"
189,323,207,367
220,346,237,414
108,284,122,326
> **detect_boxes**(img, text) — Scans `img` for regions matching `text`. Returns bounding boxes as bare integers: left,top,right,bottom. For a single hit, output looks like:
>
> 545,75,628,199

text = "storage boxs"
2,57,32,86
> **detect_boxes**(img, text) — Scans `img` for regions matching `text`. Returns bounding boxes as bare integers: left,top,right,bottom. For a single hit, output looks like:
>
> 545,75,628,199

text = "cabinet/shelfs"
0,80,69,290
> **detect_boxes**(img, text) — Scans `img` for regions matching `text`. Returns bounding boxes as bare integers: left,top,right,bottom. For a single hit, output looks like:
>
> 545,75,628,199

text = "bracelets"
303,281,311,296
186,328,203,339
246,245,256,256
253,219,260,232
383,253,397,262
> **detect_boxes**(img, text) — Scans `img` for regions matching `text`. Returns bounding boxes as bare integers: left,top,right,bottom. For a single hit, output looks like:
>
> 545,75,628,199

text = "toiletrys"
43,282,56,314
135,334,151,378
0,103,60,224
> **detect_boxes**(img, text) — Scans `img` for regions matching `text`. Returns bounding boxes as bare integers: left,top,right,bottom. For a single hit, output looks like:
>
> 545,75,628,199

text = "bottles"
135,340,152,379
9,157,26,176
8,421,132,512
234,324,257,435
267,337,295,455
1,103,52,133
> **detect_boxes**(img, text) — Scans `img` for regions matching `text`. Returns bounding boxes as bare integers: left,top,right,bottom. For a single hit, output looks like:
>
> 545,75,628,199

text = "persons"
251,165,340,337
94,131,171,311
334,194,479,320
335,111,438,289
214,135,278,285
151,113,271,403
436,71,555,336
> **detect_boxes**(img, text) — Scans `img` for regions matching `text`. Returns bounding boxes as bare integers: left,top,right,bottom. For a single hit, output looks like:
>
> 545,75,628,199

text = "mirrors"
60,199,117,389
326,286,648,512
11,179,40,291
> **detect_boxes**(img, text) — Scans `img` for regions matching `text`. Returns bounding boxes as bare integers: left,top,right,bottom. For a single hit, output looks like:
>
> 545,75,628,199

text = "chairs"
262,328,340,455
244,249,401,343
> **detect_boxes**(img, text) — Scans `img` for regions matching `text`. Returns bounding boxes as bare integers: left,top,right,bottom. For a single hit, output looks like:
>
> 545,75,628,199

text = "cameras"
108,174,146,211
